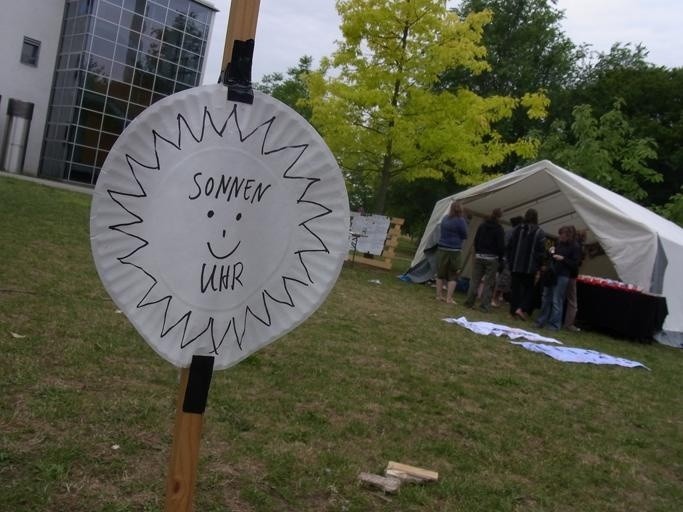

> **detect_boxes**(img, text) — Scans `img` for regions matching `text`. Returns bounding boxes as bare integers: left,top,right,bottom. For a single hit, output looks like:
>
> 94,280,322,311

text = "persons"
491,208,588,332
464,208,505,313
436,201,468,306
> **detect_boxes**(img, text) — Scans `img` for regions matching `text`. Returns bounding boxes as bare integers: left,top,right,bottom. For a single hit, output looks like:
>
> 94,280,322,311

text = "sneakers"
515,308,528,321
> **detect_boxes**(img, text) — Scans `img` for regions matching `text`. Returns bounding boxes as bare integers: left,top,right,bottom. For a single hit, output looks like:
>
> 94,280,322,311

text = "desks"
562,276,669,345
